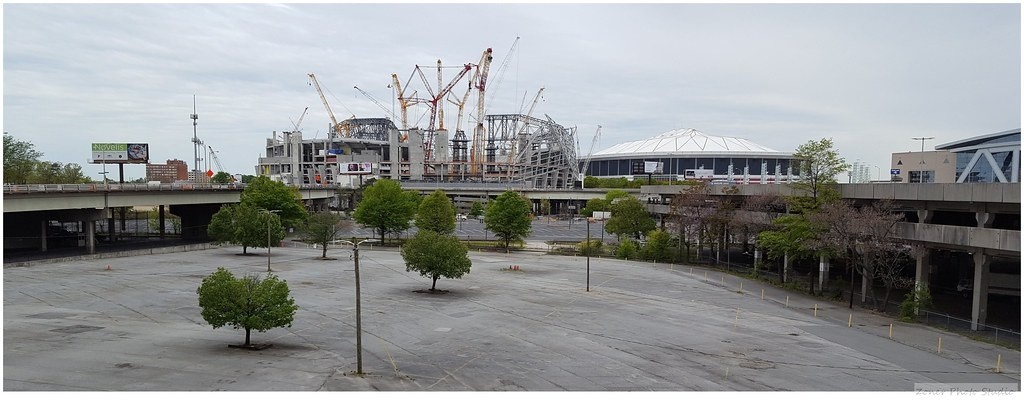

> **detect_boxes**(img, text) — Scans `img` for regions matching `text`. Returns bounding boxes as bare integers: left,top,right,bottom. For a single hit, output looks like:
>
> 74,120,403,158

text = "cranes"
289,35,602,190
